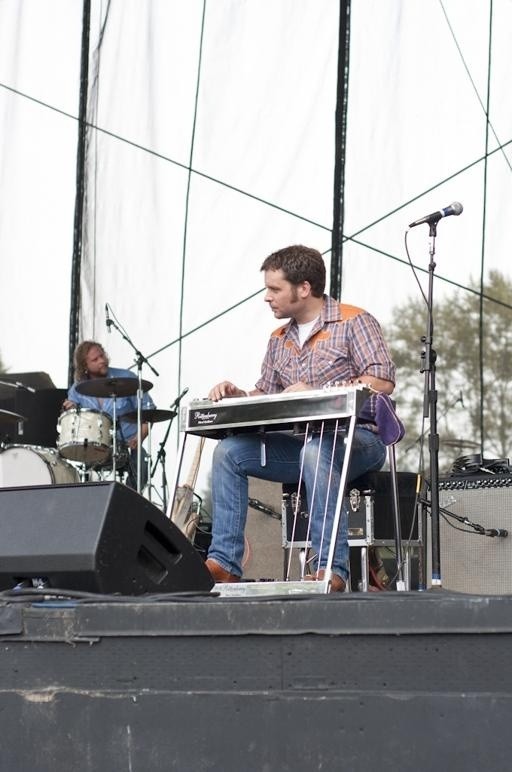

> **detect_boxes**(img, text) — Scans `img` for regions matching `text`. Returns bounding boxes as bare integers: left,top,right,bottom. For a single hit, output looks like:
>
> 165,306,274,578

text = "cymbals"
0,380,37,395
75,376,154,398
120,409,177,423
0,407,25,424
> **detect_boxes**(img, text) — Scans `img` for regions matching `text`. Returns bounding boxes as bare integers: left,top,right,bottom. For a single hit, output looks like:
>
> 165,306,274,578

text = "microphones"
481,529,508,537
105,308,111,333
170,387,189,408
409,201,463,228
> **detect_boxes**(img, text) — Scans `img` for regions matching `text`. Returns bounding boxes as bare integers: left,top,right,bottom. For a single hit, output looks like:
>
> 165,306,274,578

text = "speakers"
0,481,215,595
426,479,512,596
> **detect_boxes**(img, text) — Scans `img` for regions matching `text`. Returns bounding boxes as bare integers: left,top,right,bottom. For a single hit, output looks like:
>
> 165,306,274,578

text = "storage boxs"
281,472,422,548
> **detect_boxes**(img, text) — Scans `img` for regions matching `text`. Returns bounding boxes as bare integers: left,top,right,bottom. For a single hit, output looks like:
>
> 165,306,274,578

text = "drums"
103,440,129,470
0,443,81,488
57,407,113,465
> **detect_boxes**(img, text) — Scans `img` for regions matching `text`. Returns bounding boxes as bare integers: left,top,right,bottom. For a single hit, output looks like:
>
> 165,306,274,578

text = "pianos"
184,380,396,438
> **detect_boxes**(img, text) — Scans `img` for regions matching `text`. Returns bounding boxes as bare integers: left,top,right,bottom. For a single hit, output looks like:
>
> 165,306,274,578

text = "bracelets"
241,389,251,396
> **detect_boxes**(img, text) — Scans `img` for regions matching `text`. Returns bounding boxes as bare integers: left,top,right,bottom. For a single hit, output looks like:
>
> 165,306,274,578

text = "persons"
204,246,395,591
63,342,157,493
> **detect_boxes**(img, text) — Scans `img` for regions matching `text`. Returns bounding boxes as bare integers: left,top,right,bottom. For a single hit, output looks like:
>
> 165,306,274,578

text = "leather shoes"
205,560,239,582
304,570,345,592
